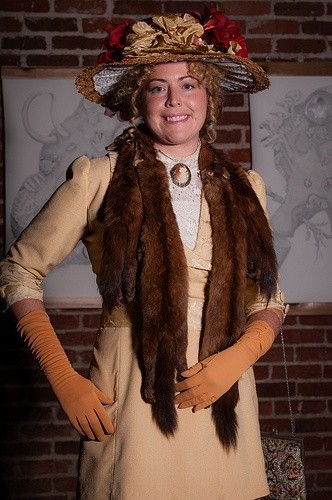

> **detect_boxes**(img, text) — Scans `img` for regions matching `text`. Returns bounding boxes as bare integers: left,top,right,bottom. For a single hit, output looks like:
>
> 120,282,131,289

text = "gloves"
172,321,275,413
15,310,114,442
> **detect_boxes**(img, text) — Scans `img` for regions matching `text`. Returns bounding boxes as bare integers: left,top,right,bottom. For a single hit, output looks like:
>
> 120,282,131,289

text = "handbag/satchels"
252,431,307,500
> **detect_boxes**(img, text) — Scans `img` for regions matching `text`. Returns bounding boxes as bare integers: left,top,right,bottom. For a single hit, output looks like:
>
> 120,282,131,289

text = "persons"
0,3,290,500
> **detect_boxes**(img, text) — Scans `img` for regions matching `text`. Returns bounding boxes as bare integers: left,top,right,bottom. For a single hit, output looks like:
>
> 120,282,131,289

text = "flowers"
90,0,248,64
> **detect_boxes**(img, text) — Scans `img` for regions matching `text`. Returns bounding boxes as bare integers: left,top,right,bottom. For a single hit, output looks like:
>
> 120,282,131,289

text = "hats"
75,0,270,120
